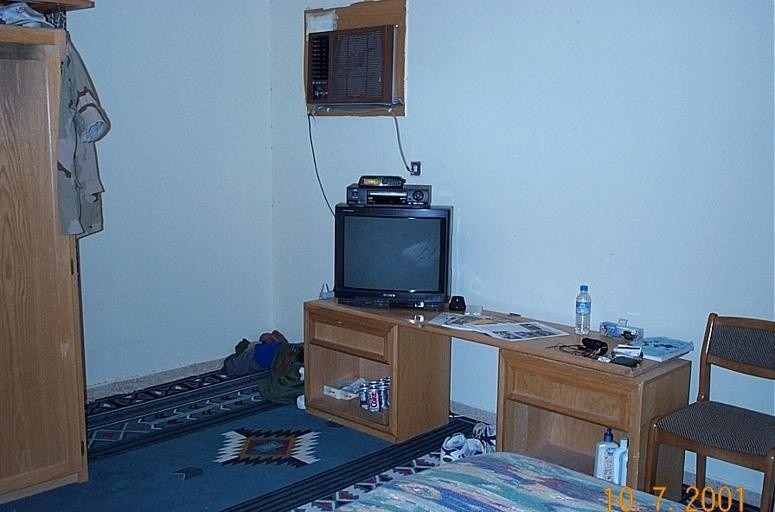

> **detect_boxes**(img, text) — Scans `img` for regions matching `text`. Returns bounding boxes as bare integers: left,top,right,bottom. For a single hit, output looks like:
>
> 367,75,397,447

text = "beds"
330,451,705,512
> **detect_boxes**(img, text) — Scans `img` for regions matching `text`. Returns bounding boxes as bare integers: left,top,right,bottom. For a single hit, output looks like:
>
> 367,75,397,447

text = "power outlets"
410,162,421,176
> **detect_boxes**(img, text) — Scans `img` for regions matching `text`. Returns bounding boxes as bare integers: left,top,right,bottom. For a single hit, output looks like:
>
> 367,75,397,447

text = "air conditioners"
305,24,397,108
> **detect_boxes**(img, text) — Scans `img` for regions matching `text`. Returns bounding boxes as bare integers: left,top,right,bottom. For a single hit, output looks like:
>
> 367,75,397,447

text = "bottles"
575,286,592,336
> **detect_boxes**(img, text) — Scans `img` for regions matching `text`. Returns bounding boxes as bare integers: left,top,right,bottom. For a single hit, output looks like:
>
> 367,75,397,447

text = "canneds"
359,376,391,413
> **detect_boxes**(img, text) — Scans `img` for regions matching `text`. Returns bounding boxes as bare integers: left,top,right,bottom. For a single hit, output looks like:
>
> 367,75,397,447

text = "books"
625,335,695,364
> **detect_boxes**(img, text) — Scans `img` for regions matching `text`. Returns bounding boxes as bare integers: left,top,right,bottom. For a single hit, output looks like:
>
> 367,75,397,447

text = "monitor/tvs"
334,202,453,313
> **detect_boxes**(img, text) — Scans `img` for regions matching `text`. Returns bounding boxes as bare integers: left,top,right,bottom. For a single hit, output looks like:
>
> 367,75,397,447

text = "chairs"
640,313,774,512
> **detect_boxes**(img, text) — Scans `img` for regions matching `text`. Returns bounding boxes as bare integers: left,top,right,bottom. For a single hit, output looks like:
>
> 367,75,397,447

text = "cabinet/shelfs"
2,23,92,509
303,297,693,503
